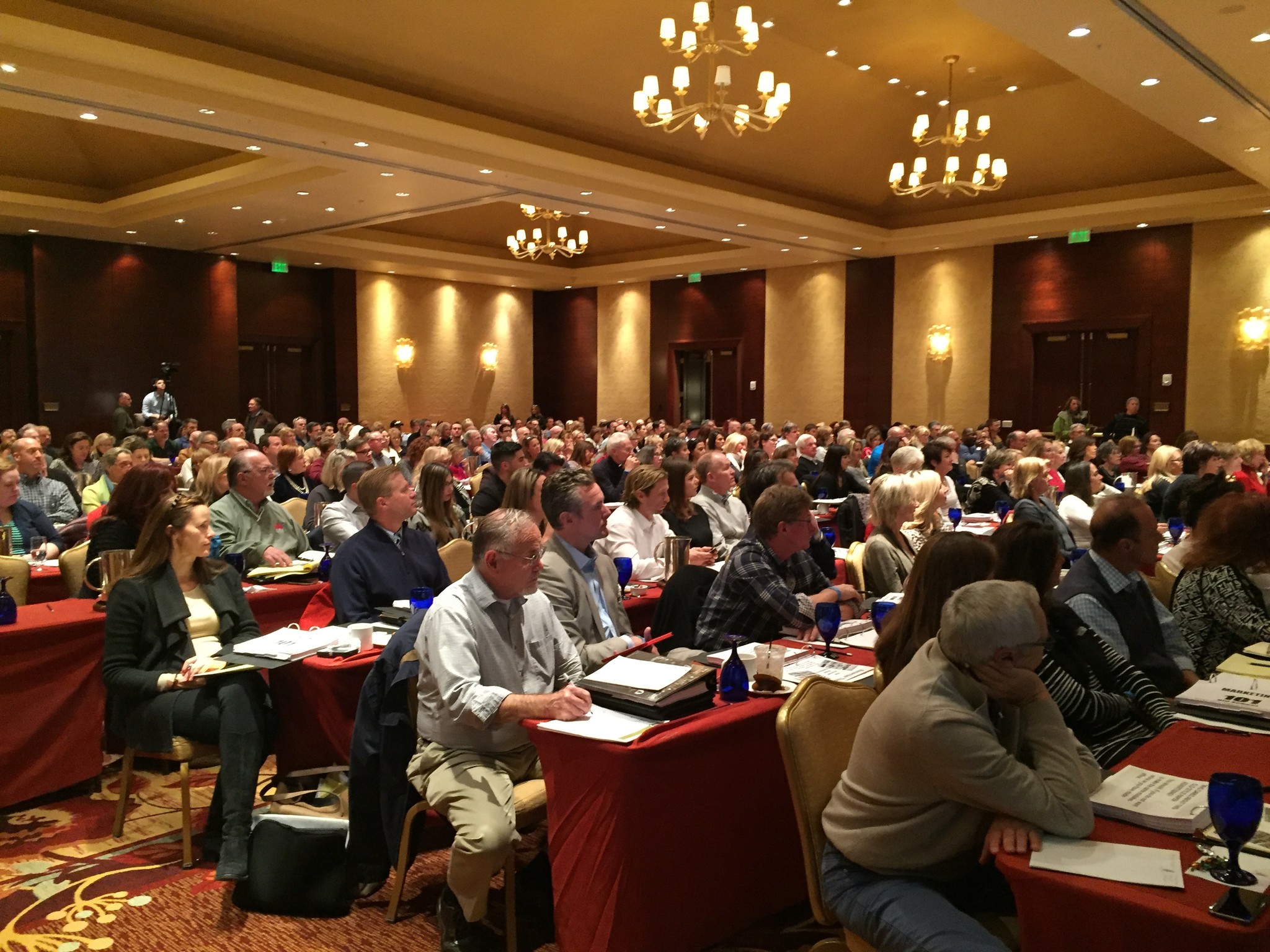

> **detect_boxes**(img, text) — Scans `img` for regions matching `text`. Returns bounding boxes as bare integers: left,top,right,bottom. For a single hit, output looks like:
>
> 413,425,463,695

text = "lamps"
631,0,790,145
891,59,1007,208
503,200,592,259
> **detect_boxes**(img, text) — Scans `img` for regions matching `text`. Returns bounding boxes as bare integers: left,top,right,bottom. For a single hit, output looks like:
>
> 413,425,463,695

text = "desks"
0,503,1269,947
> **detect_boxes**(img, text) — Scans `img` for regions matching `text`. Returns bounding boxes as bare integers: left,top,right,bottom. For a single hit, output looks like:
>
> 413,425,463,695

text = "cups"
814,602,841,660
721,653,757,682
945,522,954,532
1070,549,1087,568
348,622,375,652
754,644,787,686
871,601,897,638
410,584,433,610
225,552,246,575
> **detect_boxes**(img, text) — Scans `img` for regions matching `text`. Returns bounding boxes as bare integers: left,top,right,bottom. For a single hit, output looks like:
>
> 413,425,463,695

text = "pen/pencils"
187,663,194,669
856,590,873,594
563,672,593,715
806,646,852,657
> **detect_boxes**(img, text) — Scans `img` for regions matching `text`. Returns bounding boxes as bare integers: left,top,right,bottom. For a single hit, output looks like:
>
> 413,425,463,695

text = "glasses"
791,517,812,524
369,438,386,443
1132,444,1143,448
445,479,457,487
501,430,512,432
202,440,218,446
1170,457,1182,462
355,448,373,454
532,404,539,407
492,545,546,562
1015,637,1056,653
1106,452,1122,456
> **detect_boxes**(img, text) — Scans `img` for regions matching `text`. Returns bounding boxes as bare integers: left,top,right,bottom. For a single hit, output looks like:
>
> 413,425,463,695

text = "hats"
389,420,404,428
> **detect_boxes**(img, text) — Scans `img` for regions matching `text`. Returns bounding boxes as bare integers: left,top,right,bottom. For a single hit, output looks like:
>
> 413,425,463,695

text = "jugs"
654,535,694,583
0,573,19,626
83,548,140,603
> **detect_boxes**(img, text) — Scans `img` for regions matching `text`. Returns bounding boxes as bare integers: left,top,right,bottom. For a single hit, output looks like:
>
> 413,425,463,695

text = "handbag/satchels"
228,765,355,918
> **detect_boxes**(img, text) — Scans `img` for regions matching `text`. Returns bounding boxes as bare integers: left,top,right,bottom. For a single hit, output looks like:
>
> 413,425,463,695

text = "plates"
747,679,798,694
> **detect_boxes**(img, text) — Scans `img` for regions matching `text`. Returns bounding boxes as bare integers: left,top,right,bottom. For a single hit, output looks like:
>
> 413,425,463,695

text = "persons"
0,377,1270,951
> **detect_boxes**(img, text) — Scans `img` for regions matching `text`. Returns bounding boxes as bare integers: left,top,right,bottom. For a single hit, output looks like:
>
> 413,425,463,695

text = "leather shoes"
436,886,471,951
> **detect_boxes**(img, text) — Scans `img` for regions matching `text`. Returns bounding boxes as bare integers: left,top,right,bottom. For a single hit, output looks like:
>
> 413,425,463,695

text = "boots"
196,731,266,880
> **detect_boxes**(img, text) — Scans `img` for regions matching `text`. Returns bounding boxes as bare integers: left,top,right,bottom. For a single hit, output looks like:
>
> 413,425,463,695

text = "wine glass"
318,542,336,583
31,536,47,573
1205,770,1263,888
1168,518,1184,549
995,501,1010,521
720,633,751,702
821,527,836,549
613,556,634,600
948,507,962,532
